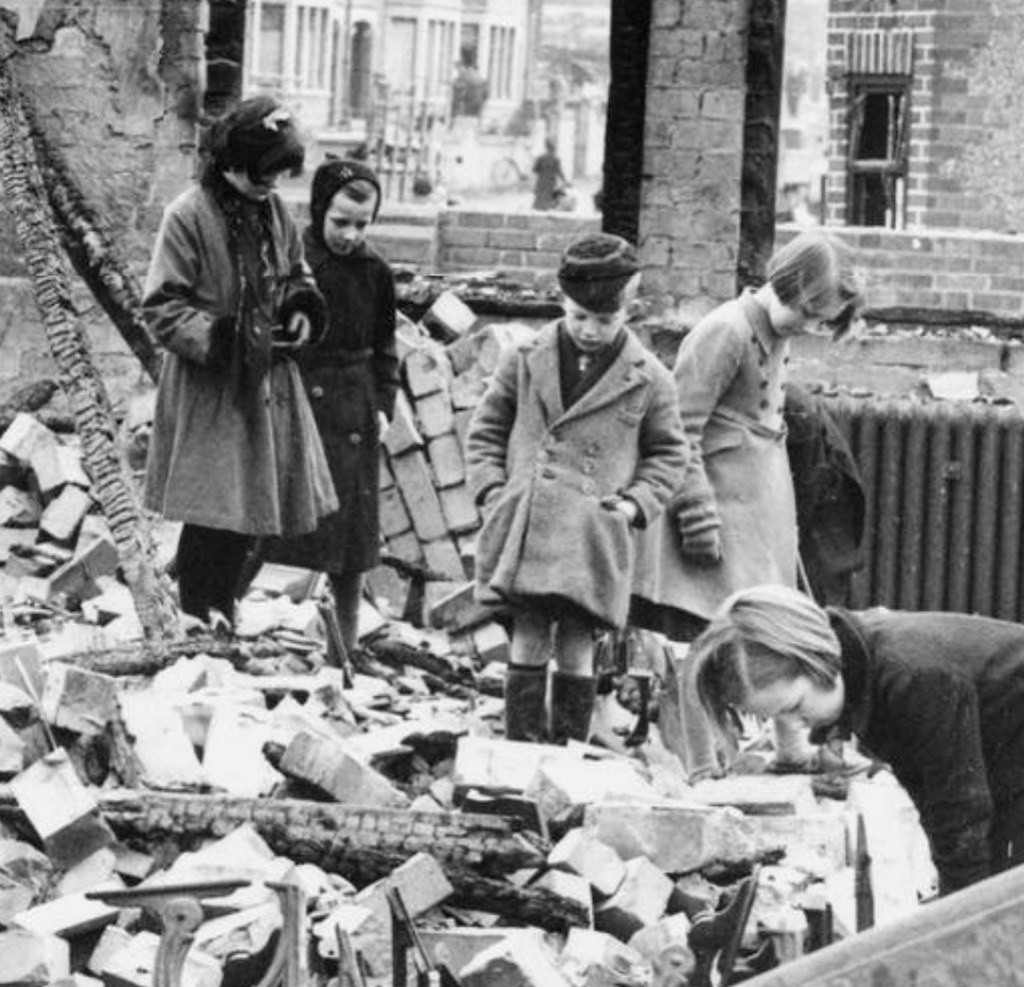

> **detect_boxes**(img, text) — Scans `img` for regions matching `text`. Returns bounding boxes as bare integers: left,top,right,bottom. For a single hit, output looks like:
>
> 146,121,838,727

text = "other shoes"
771,743,860,777
328,644,397,683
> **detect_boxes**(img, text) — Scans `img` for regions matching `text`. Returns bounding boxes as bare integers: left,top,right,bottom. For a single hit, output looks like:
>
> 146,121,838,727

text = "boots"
505,663,602,748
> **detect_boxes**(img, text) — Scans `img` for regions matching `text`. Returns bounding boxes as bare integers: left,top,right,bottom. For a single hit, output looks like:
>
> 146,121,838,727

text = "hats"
557,229,640,313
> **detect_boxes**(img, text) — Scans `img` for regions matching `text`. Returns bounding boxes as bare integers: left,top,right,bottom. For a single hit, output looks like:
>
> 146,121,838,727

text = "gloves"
674,495,724,567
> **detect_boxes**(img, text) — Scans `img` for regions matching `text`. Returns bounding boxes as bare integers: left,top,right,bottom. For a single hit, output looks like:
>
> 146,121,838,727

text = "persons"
134,94,400,676
629,230,868,638
462,234,688,747
678,585,1024,901
